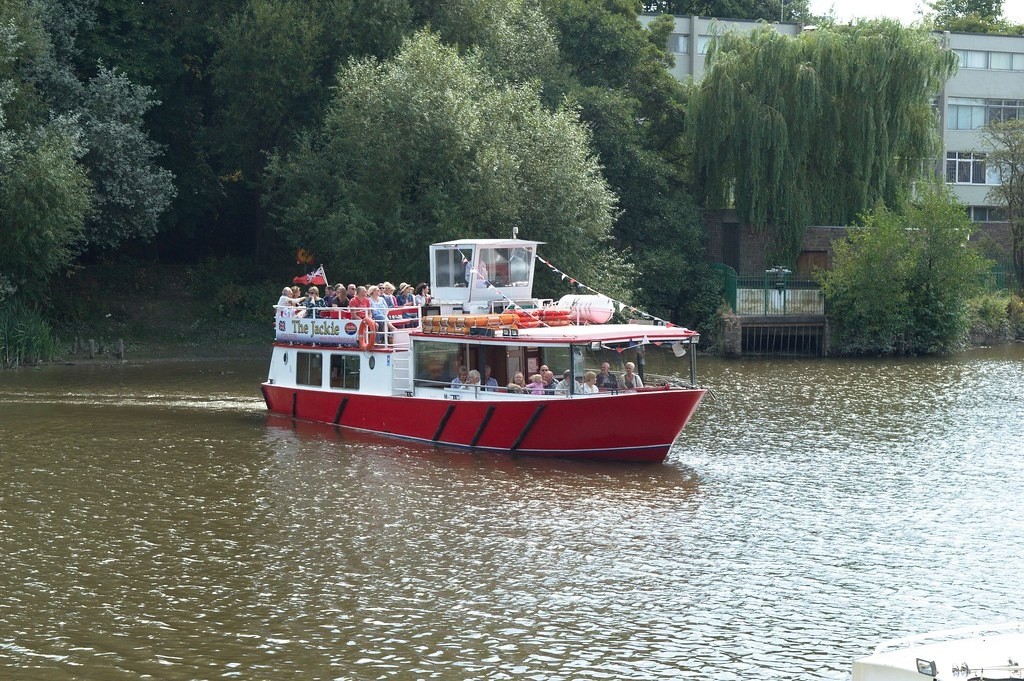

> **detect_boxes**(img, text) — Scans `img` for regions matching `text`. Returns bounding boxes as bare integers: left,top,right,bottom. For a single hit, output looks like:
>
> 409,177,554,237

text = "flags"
293,267,326,285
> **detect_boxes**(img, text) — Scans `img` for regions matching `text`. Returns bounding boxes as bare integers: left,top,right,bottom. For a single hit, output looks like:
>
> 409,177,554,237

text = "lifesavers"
358,317,377,349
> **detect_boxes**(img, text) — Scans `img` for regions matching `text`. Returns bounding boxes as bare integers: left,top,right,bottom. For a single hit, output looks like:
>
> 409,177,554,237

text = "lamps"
503,328,510,337
588,340,602,351
510,329,518,337
513,227,519,239
688,335,701,344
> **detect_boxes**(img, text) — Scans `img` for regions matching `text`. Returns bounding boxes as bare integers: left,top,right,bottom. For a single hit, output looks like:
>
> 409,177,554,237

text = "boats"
259,226,708,464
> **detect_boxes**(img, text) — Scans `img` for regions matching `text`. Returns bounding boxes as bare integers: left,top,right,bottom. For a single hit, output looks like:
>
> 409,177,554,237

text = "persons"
595,362,617,388
277,251,645,395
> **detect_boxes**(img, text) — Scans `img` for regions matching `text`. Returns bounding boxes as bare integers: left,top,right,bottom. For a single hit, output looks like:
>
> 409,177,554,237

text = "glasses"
542,369,548,371
380,288,384,289
308,292,314,294
350,288,356,290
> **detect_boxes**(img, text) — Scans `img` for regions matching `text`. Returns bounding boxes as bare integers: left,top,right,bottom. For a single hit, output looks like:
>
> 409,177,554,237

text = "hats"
400,283,411,293
369,286,377,295
